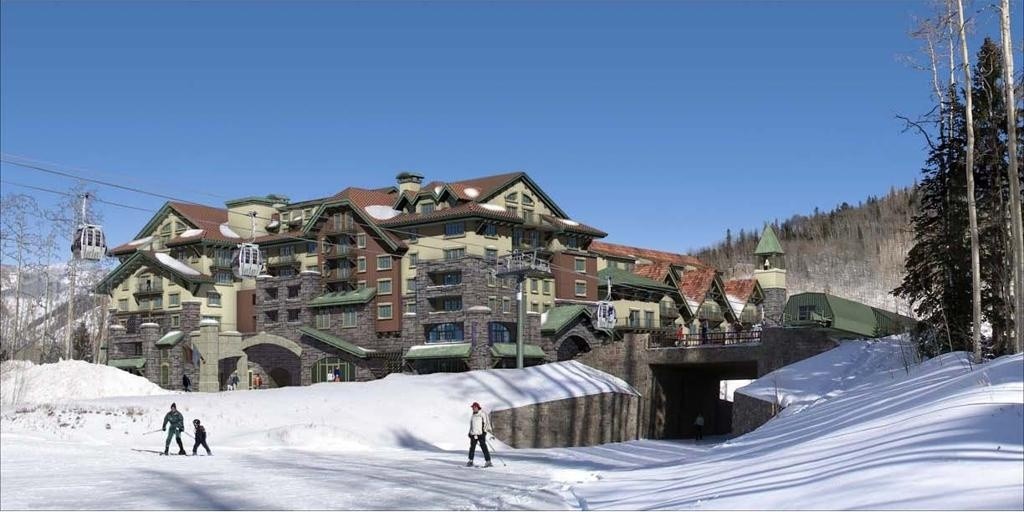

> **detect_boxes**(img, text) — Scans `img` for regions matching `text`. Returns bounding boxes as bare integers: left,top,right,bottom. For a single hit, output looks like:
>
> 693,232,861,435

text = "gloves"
162,426,165,431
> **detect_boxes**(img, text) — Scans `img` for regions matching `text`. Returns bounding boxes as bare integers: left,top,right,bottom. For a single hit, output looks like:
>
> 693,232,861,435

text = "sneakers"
164,450,168,455
485,461,491,467
467,461,473,467
178,450,185,454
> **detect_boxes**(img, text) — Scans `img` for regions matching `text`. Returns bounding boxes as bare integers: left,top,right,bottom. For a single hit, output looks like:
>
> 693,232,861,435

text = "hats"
170,402,176,409
470,402,482,410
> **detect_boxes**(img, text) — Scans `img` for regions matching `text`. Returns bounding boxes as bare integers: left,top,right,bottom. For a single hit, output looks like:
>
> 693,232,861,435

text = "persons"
675,323,684,340
192,419,213,456
227,372,240,392
466,402,492,468
255,373,262,389
694,413,704,440
699,317,708,344
327,366,342,382
182,374,191,392
733,320,744,343
162,403,186,456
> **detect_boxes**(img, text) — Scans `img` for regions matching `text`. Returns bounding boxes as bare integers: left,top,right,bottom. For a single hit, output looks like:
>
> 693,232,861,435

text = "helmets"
193,419,200,426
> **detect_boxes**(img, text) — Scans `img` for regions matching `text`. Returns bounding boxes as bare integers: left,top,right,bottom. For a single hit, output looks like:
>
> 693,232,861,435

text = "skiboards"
465,461,495,468
159,451,188,456
